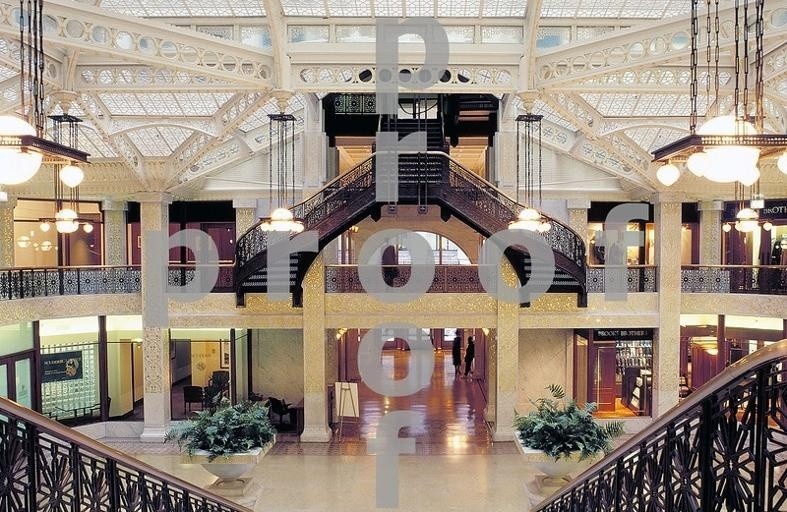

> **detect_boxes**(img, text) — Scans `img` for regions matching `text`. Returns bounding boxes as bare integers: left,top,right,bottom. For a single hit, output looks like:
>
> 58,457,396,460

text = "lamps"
0,0,92,186
39,114,96,236
259,112,304,232
511,113,552,231
722,165,773,232
651,2,787,185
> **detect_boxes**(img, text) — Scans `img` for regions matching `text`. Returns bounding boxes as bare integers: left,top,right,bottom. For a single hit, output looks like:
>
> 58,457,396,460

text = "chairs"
183,371,229,416
268,397,295,425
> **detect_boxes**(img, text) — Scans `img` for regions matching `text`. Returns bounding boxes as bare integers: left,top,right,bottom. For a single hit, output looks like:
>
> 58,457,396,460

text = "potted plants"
512,384,626,494
163,401,278,495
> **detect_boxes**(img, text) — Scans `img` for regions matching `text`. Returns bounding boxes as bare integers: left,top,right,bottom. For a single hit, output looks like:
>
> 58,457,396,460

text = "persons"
462,336,477,378
450,330,462,377
